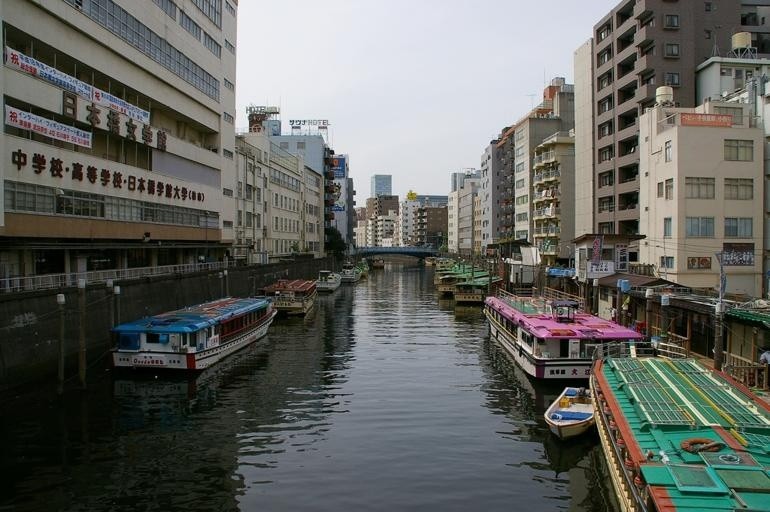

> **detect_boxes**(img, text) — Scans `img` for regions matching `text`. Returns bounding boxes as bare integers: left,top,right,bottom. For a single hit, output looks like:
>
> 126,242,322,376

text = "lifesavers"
682,438,720,452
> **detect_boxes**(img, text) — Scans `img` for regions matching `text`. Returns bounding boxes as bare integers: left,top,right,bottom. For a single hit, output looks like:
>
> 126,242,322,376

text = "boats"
315,256,386,293
588,355,770,512
108,294,278,371
482,295,650,381
543,386,596,441
255,278,318,316
434,255,504,303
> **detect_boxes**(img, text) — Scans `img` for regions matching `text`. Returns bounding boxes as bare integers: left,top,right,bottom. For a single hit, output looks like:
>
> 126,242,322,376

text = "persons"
759,347,770,389
611,305,617,321
